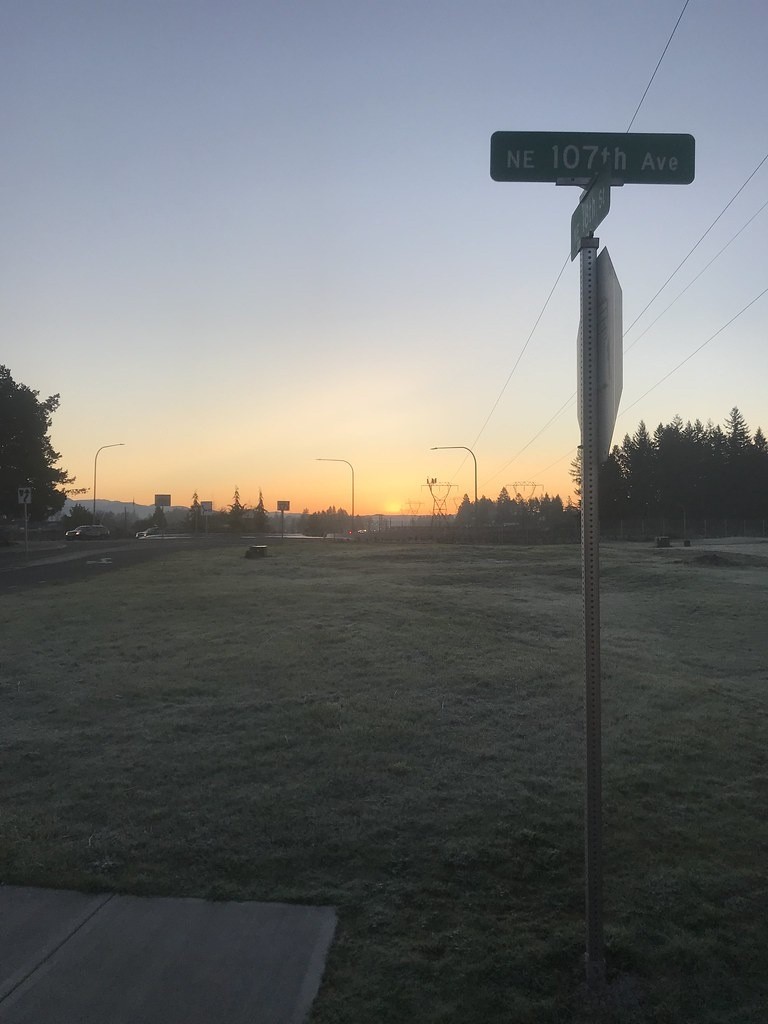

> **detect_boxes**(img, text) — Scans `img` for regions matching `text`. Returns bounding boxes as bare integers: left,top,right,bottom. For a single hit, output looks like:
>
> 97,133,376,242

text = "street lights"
429,445,477,527
93,443,126,524
316,459,359,534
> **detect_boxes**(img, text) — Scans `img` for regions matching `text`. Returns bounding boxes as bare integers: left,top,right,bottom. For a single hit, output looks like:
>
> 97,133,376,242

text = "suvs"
135,526,161,540
66,524,110,542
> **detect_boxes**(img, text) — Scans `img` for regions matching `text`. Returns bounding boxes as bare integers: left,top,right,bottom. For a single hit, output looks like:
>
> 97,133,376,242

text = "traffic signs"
487,129,696,186
571,183,611,264
